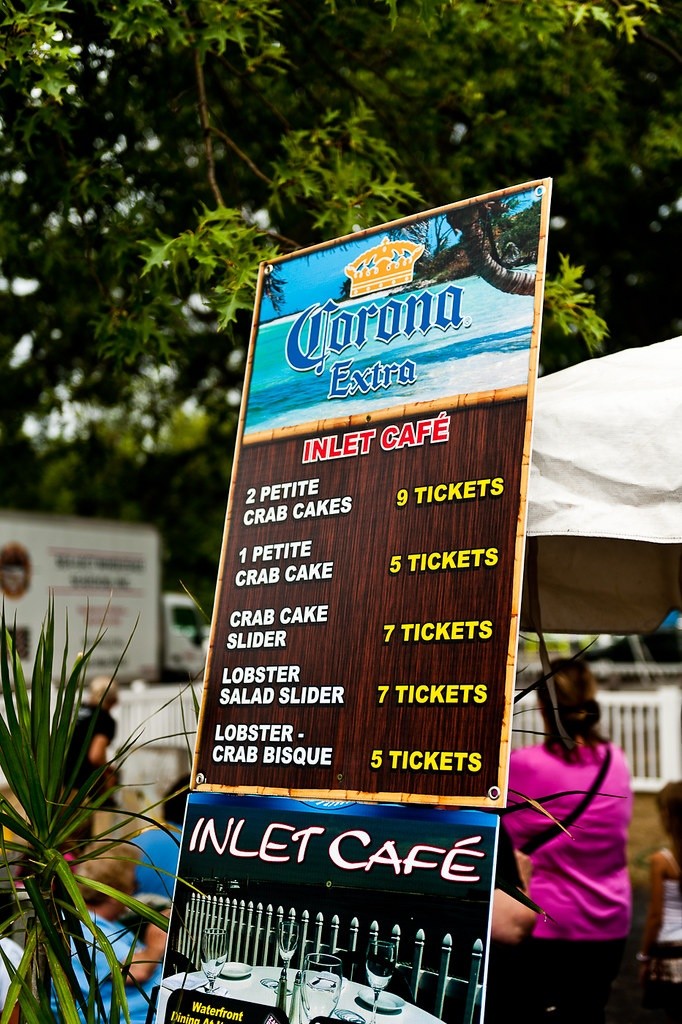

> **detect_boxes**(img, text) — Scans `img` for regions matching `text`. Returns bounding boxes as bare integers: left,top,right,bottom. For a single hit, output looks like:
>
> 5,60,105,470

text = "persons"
635,781,682,1024
503,658,637,1024
0,546,536,1024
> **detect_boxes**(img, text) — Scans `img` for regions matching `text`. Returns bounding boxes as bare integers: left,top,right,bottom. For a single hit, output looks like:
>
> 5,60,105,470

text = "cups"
300,953,342,1024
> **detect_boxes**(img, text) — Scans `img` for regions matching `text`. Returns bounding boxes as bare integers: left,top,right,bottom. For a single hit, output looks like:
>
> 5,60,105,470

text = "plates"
220,962,253,979
358,989,406,1012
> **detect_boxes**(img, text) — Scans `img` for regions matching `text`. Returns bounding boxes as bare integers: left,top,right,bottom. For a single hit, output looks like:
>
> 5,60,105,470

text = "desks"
157,966,446,1024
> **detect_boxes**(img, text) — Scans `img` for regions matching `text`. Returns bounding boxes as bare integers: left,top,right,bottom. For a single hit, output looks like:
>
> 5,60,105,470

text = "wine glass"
199,928,228,996
363,941,396,1024
273,921,299,997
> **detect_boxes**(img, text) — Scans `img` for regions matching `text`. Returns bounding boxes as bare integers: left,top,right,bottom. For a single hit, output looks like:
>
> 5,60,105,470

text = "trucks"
1,512,213,685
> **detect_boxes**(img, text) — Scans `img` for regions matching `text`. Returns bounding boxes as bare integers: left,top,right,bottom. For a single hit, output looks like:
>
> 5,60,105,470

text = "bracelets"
636,951,649,962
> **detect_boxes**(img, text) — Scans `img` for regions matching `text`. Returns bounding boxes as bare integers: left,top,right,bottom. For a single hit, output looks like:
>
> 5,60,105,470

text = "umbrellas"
513,336,682,636
